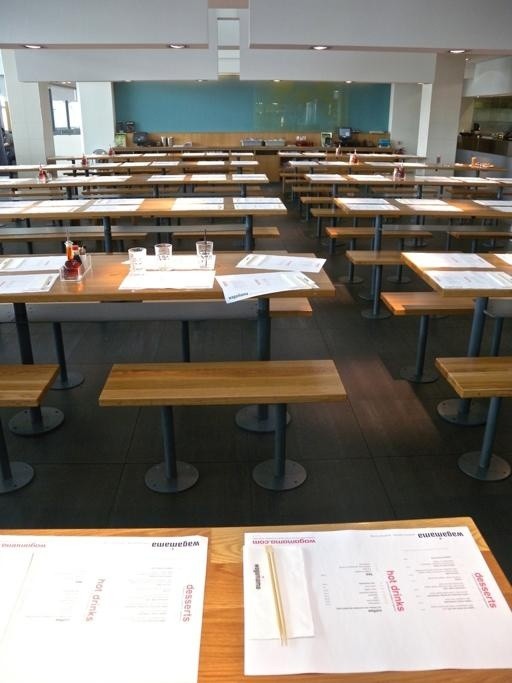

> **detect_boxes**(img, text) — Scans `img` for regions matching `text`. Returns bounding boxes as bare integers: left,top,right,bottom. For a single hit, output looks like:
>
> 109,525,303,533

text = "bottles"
63,240,87,261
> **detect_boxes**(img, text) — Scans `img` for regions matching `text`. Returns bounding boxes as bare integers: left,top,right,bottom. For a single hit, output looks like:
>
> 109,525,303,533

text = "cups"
128,247,147,275
154,243,172,271
196,241,213,271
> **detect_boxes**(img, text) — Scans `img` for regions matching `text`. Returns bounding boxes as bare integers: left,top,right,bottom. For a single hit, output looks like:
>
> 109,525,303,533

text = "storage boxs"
264,140,287,148
241,140,263,147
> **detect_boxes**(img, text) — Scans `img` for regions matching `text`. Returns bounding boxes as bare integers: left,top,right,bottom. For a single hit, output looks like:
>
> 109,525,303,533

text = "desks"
0,146,512,683
